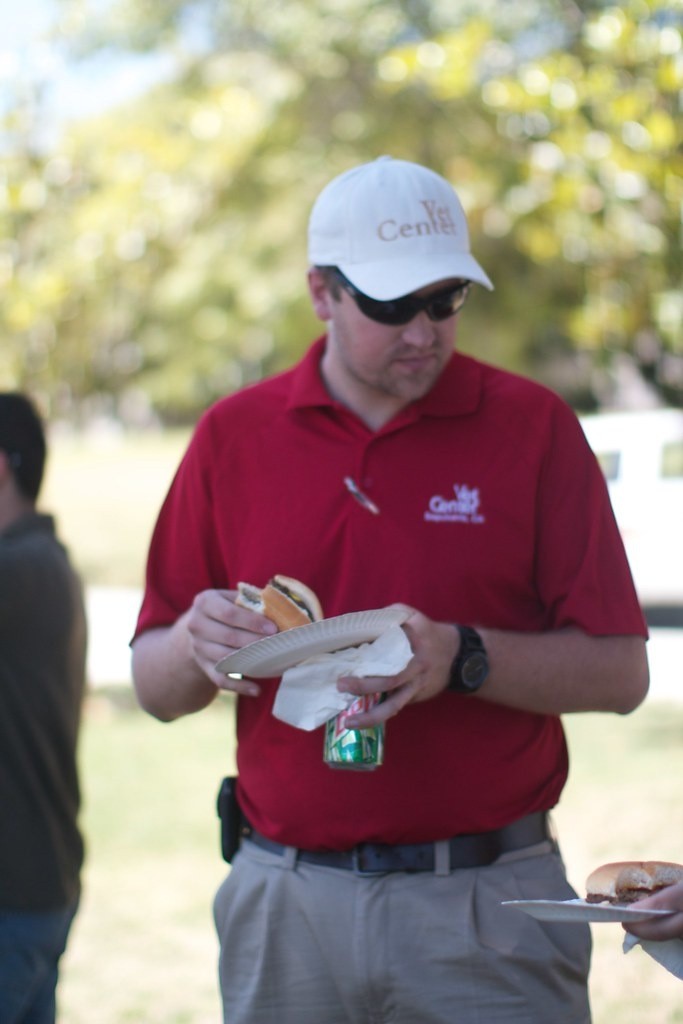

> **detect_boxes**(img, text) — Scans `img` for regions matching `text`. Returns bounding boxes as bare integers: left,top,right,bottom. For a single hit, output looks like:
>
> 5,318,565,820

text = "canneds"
322,690,387,772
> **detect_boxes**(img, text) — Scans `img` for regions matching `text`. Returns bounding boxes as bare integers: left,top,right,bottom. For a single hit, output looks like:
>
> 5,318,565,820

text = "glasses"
335,269,469,325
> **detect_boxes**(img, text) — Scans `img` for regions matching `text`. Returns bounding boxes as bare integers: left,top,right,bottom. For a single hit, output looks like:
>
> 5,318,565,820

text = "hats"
308,155,495,302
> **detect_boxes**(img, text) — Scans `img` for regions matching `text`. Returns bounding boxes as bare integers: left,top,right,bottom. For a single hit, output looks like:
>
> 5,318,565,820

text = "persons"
129,155,651,1024
0,390,87,1024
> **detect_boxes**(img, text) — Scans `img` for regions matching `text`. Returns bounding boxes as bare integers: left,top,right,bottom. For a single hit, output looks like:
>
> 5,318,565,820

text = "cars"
572,407,683,615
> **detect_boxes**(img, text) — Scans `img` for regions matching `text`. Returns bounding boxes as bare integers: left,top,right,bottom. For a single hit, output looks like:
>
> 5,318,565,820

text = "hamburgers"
234,574,323,632
585,861,683,908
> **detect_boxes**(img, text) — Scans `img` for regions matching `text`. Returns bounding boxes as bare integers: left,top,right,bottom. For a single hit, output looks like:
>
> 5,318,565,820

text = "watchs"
449,624,489,691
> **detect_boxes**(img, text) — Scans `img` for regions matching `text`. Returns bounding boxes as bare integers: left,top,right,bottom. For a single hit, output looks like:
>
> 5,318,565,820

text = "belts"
241,809,549,877
